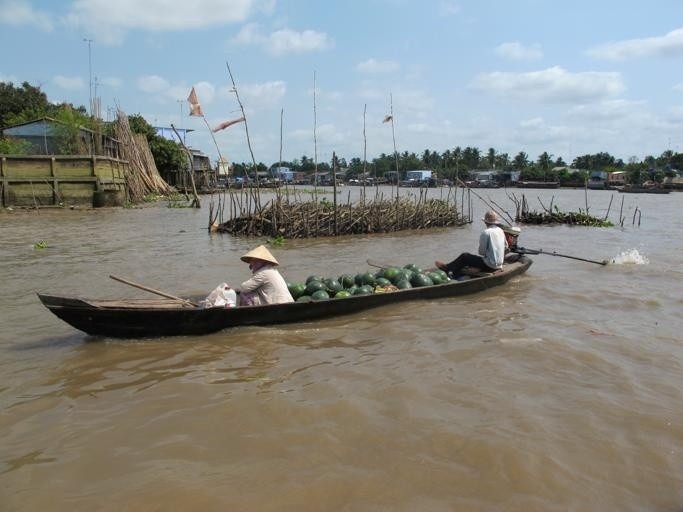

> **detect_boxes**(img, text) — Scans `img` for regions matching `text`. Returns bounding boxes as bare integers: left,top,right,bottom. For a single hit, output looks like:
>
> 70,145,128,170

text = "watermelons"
288,263,447,301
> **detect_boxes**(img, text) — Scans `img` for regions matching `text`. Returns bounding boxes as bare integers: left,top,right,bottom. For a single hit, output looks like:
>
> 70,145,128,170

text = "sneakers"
435,260,448,273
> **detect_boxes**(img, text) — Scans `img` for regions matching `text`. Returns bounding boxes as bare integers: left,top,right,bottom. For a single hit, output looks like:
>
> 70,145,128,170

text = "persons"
435,210,507,278
239,248,295,307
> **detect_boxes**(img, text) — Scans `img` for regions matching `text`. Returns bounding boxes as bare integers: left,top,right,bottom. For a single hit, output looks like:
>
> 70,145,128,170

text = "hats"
481,210,501,225
240,244,279,265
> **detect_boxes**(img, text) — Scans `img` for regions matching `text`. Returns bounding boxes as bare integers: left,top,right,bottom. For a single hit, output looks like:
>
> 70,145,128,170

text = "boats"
39,258,544,338
